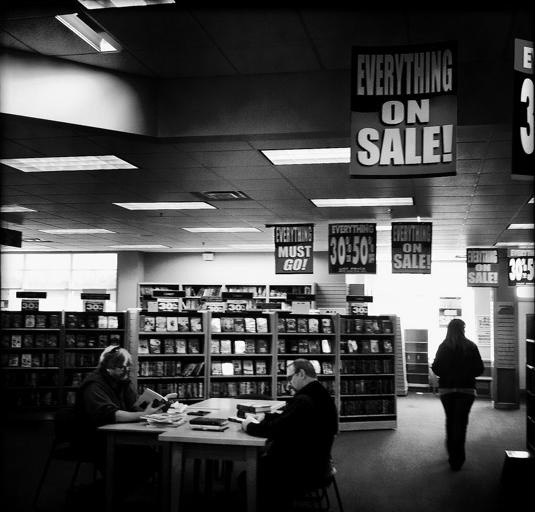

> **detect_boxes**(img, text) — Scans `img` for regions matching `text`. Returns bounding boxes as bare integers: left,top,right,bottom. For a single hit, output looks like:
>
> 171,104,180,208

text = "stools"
316,467,344,512
505,450,535,483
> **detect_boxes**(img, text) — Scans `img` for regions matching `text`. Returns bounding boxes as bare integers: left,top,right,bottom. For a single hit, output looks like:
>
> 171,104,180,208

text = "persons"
71,346,166,511
234,358,340,510
430,318,486,472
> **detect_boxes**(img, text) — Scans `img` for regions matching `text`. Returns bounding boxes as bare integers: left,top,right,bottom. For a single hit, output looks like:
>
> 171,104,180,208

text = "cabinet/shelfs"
403,328,431,387
1,309,64,421
275,312,340,418
133,310,207,402
525,313,535,452
63,311,130,416
337,313,397,429
207,309,276,405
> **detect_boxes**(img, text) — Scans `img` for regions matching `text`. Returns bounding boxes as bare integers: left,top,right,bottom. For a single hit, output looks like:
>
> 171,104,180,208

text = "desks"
159,413,269,512
105,431,169,512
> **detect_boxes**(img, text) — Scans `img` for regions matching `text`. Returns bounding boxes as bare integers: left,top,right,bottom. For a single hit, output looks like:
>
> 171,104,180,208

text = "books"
210,318,269,396
2,312,124,417
340,318,395,415
138,315,204,399
278,318,336,396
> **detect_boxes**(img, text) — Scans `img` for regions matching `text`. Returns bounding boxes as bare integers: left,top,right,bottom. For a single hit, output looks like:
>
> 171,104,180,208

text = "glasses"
288,371,299,381
